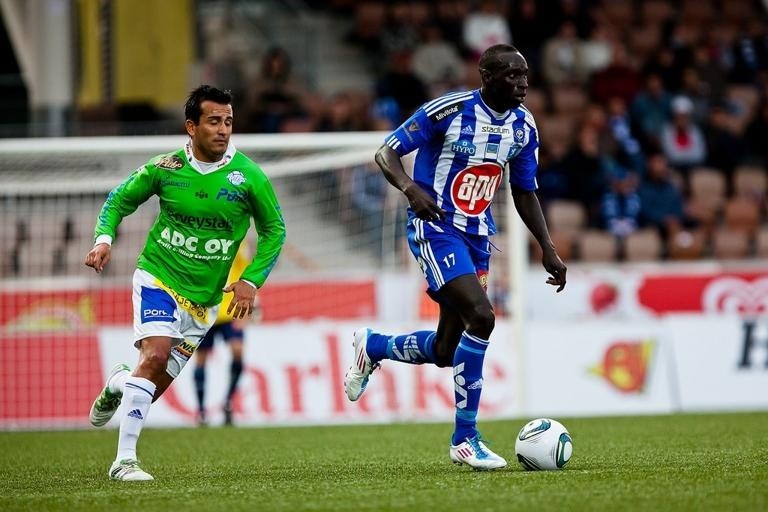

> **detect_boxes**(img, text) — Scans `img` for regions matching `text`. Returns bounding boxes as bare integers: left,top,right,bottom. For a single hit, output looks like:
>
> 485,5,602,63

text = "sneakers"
89,363,130,427
344,327,378,401
109,459,154,482
450,432,507,471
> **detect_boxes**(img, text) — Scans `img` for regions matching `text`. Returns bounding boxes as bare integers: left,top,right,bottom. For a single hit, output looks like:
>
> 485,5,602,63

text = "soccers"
515,418,573,470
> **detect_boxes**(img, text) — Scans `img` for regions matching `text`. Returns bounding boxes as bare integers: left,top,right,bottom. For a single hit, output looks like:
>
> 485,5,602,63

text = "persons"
341,41,569,473
372,0,767,234
248,74,373,134
84,81,287,483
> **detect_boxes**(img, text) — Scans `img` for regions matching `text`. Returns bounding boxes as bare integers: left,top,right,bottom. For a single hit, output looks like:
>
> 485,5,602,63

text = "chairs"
528,0,768,265
